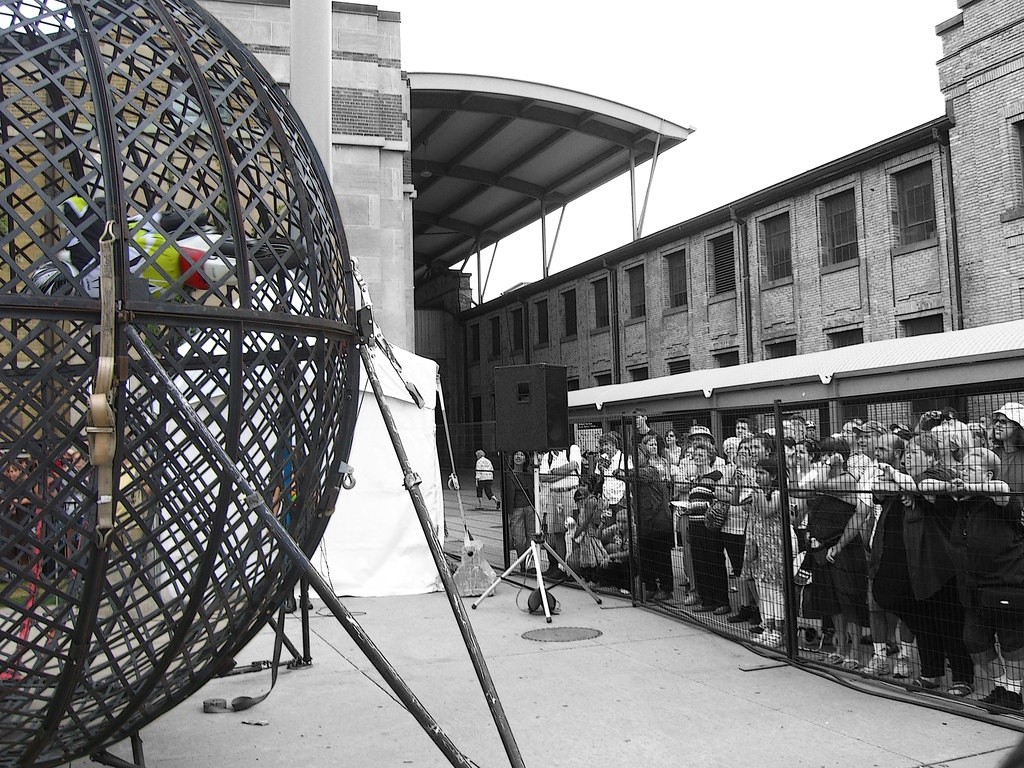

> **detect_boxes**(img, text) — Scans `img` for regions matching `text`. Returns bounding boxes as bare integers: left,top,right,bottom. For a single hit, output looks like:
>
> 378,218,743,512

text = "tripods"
471,452,602,623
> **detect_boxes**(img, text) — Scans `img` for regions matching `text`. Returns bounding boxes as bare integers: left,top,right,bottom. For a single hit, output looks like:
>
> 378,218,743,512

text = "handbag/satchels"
704,466,739,532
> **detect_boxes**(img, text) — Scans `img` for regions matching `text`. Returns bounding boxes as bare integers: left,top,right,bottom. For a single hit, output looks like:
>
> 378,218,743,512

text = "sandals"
905,677,941,690
946,679,975,697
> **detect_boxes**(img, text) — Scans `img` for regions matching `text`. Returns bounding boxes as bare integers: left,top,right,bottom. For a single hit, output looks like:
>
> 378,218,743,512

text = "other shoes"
979,687,1022,714
496,501,500,510
647,581,783,647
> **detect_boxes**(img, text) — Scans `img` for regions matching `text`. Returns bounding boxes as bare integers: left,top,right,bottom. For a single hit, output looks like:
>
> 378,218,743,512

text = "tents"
140,268,449,607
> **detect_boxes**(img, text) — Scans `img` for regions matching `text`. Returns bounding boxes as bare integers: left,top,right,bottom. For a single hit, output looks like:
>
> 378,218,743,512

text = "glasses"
665,433,676,437
994,419,1012,423
940,415,957,421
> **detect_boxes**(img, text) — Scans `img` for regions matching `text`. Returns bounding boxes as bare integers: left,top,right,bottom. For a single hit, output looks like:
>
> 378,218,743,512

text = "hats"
806,420,816,428
574,489,588,499
889,423,910,432
993,402,1024,429
853,421,888,436
688,426,715,446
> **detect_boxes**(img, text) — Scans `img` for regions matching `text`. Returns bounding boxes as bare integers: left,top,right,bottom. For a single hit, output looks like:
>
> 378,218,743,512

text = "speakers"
494,363,570,452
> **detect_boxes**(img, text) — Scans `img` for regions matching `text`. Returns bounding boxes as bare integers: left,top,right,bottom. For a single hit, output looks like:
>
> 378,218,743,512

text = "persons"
25,195,206,323
502,402,1024,715
475,450,500,510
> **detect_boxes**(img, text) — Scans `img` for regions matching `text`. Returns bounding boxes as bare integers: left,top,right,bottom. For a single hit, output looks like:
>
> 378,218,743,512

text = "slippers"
826,652,864,669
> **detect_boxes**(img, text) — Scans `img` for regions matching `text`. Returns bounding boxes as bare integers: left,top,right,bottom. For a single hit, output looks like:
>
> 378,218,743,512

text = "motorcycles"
48,201,306,287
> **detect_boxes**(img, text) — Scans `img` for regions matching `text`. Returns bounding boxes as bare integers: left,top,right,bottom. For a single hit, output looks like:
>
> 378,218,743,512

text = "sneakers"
861,658,891,677
894,653,914,676
